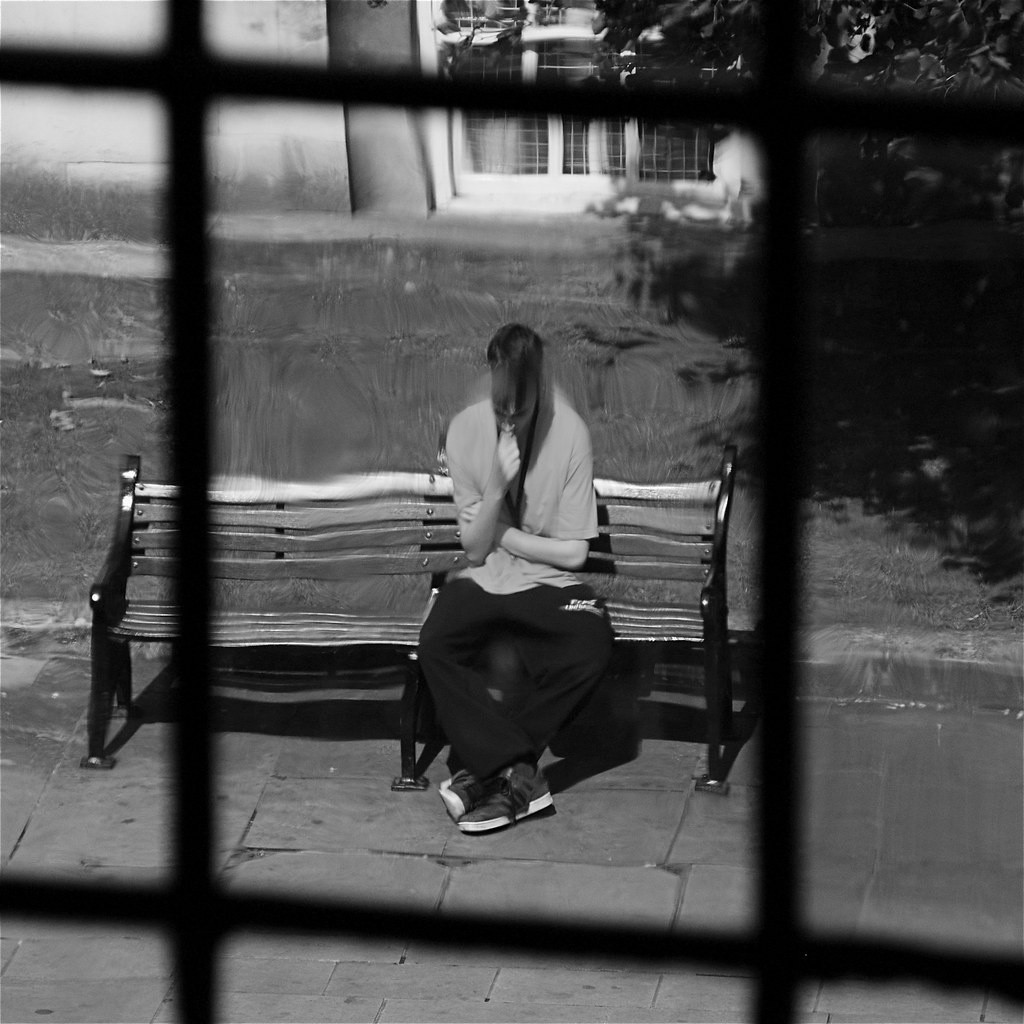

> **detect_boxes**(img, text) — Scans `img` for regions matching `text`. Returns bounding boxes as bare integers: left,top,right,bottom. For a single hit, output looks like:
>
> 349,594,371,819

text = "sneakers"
458,760,553,832
438,767,488,820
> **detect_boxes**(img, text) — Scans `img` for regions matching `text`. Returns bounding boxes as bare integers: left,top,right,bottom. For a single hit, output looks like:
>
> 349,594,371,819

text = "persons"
417,322,614,832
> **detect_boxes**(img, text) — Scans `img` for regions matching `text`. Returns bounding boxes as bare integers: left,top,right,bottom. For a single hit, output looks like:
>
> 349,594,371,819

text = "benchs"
79,432,738,795
